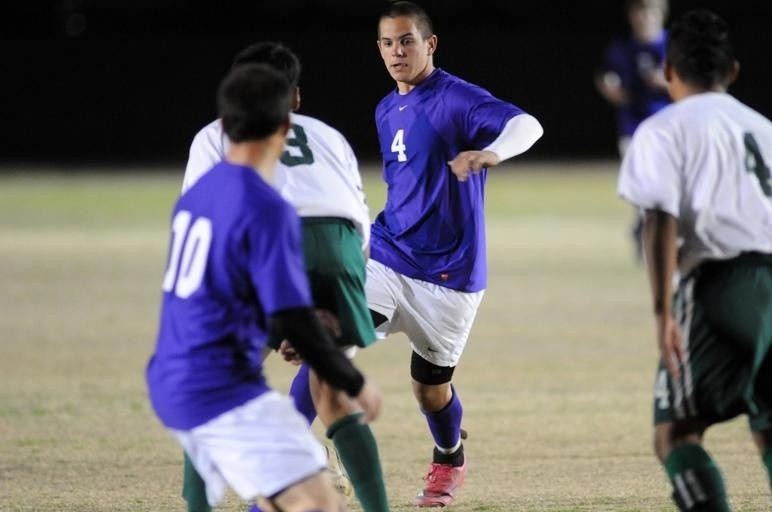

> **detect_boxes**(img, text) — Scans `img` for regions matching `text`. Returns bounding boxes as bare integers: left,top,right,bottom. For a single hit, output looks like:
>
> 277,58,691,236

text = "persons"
179,38,391,510
144,65,385,512
247,2,548,512
616,11,772,510
590,1,675,269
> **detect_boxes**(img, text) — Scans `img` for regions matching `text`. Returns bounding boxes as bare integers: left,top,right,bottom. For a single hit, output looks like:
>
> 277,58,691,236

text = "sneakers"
417,452,467,508
